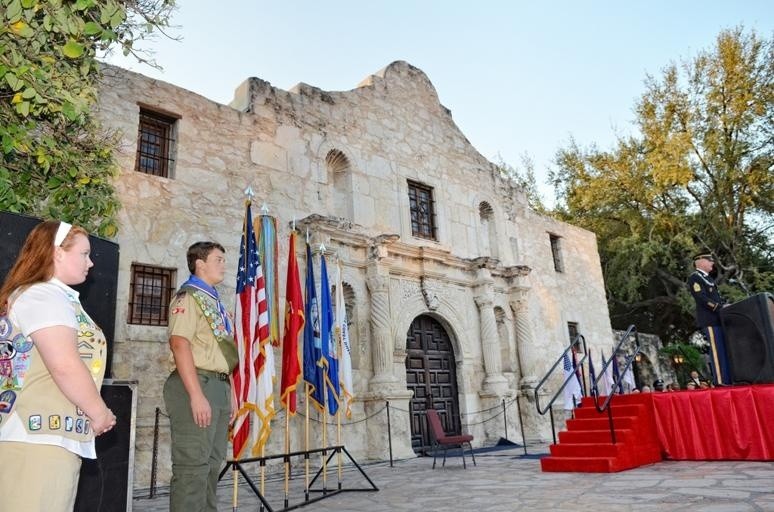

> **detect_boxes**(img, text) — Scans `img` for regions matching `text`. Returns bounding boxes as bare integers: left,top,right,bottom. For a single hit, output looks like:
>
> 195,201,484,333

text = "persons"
688,251,734,387
163,242,239,512
632,370,709,393
0,222,117,512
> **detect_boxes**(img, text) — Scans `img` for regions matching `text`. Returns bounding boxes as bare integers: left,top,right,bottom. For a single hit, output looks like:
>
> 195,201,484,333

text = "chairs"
425,408,476,469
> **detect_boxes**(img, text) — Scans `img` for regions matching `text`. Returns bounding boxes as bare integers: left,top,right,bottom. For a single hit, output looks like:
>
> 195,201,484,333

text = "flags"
563,346,636,410
229,200,354,461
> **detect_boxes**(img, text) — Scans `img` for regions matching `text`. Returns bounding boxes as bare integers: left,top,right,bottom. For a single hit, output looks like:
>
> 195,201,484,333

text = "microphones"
728,279,744,285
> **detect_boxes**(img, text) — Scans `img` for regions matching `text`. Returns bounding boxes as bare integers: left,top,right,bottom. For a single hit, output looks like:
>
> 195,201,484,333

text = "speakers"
0,210,120,379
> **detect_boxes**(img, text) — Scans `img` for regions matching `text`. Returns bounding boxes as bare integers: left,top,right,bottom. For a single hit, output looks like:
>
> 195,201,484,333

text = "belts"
195,368,230,384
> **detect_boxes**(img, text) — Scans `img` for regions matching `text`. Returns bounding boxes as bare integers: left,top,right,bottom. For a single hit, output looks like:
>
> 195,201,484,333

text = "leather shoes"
714,383,733,388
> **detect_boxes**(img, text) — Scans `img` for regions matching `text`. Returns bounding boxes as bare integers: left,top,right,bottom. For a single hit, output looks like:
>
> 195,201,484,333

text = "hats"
692,251,716,268
653,379,664,387
